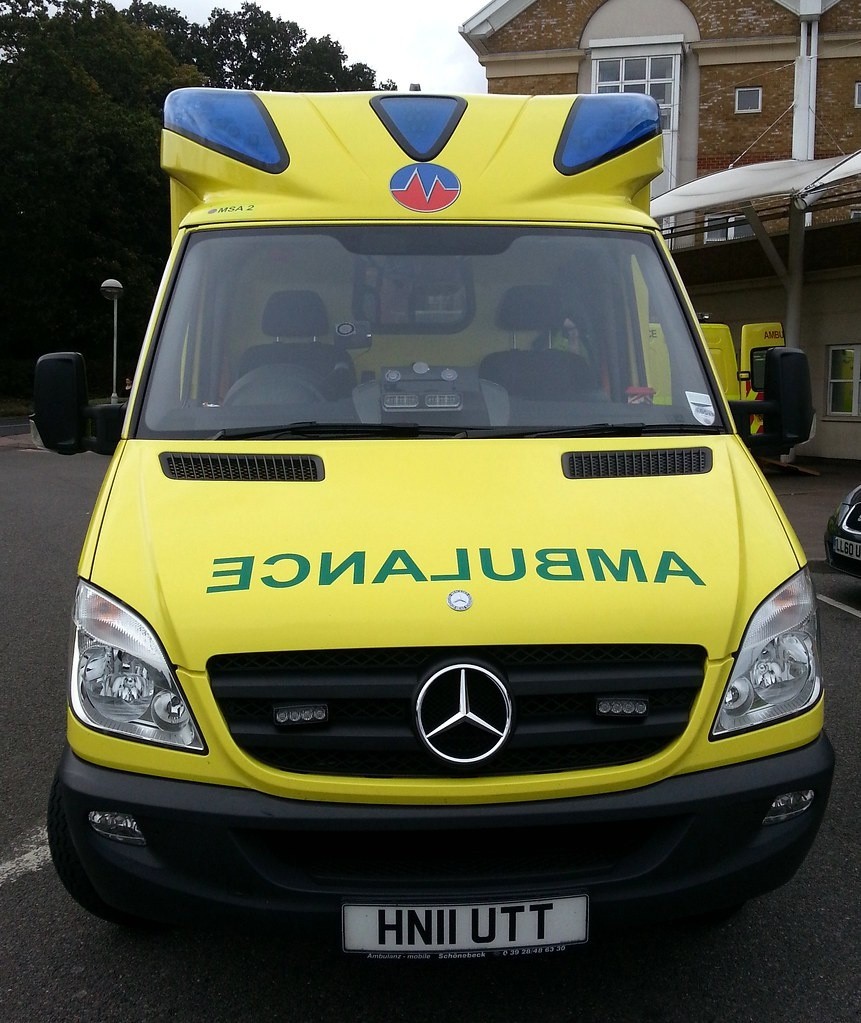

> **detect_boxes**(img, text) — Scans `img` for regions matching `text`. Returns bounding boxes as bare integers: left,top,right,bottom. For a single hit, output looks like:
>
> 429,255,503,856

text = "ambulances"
29,80,837,964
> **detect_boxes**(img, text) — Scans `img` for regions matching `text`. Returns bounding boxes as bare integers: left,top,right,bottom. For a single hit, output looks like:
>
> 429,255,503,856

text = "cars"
824,484,861,580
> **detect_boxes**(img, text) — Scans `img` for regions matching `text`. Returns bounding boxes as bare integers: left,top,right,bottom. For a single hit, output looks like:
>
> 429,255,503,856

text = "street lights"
99,279,125,405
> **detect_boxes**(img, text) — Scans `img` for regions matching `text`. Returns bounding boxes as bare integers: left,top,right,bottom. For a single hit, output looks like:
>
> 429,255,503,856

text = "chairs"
478,285,607,403
236,290,356,401
